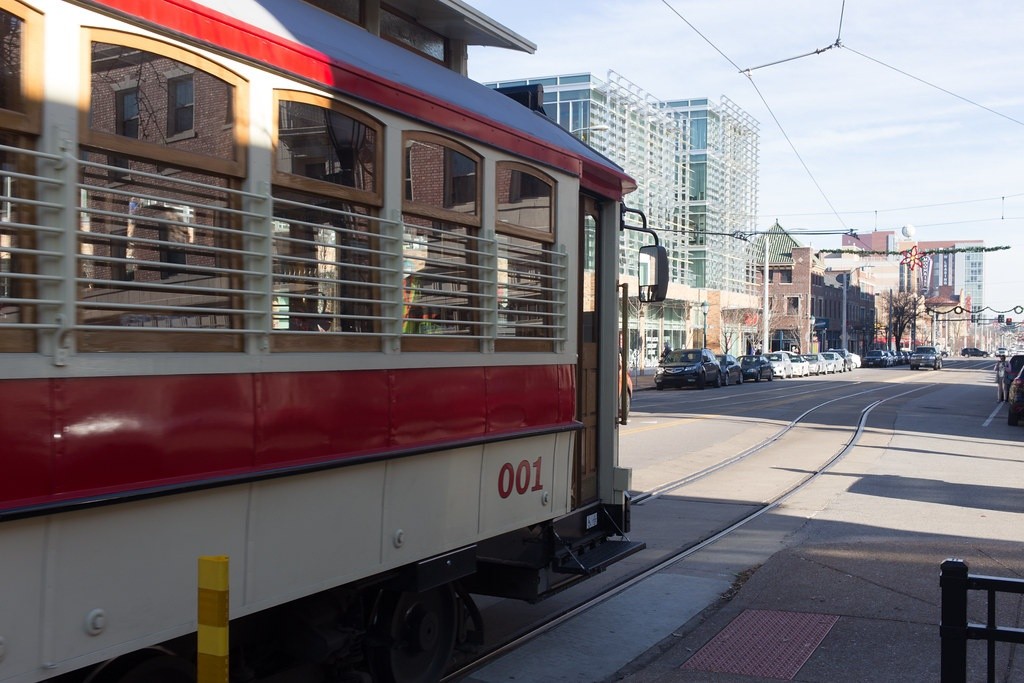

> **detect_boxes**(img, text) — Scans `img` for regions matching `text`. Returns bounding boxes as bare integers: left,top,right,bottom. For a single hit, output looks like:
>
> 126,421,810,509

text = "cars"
1002,355,1024,402
961,347,988,358
736,355,774,383
1006,365,1024,426
771,348,861,377
995,347,1024,357
715,354,744,387
939,350,949,359
883,350,914,367
758,352,794,379
860,350,888,369
618,360,634,415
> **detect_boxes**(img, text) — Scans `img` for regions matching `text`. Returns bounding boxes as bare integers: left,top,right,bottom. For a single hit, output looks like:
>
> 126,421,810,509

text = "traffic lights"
1005,318,1012,325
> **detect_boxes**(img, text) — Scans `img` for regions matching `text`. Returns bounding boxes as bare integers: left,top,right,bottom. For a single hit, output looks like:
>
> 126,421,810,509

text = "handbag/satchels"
1005,378,1011,386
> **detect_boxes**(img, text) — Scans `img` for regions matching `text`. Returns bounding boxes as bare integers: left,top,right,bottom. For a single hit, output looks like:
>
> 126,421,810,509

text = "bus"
1,0,671,683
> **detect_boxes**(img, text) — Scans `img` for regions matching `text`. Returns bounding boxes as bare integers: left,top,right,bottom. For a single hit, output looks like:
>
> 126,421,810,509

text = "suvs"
654,347,722,391
909,345,943,371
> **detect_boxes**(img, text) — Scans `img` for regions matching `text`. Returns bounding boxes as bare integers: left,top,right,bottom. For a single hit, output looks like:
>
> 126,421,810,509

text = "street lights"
888,285,912,351
700,299,711,348
809,314,816,352
762,227,810,353
840,264,875,350
933,300,952,346
912,287,928,350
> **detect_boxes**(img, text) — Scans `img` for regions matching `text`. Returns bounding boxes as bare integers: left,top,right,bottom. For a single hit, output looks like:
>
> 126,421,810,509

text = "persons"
661,342,671,357
994,355,1012,403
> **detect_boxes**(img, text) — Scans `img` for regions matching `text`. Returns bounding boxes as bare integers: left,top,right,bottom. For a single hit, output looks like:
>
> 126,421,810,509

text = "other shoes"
997,399,1002,403
1003,400,1008,403
